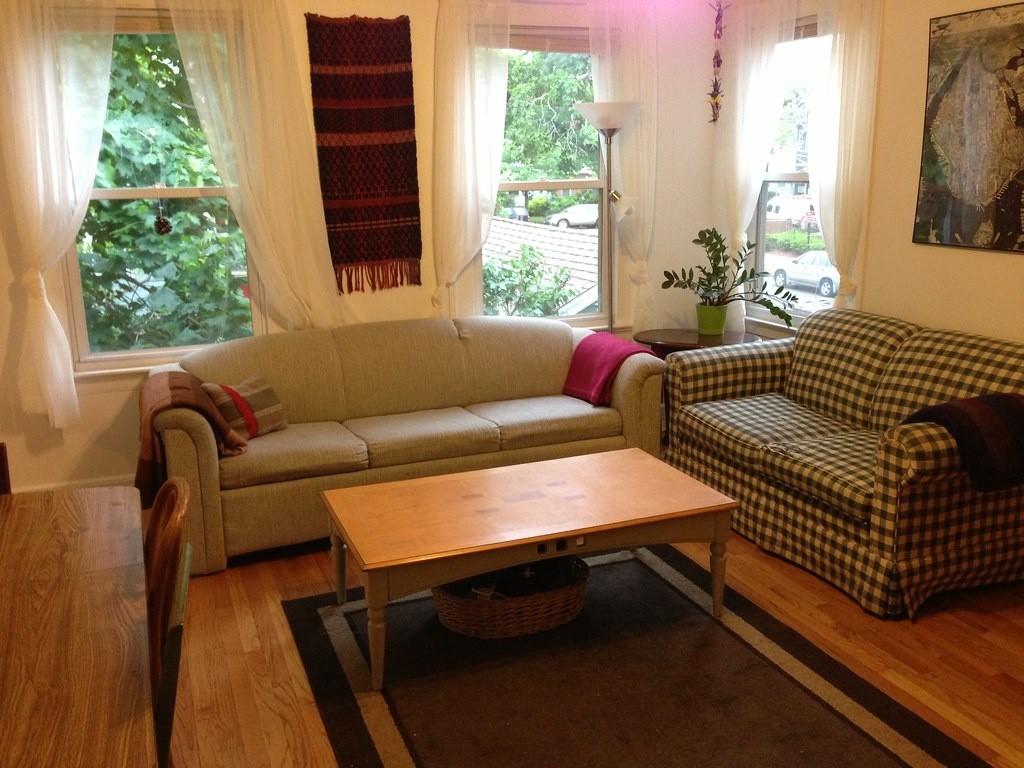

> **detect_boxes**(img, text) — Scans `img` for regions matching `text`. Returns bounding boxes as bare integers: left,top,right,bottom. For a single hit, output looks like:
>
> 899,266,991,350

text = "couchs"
134,317,669,577
665,306,1024,616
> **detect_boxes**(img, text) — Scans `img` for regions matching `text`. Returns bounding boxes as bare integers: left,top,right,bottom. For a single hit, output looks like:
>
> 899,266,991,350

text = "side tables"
633,327,762,361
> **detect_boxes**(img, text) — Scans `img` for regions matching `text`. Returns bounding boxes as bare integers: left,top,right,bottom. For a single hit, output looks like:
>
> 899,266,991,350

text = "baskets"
431,559,589,638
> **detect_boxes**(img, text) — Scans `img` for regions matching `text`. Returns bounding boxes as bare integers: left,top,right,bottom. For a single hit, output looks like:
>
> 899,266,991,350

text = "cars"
773,249,840,297
544,203,599,229
503,206,531,222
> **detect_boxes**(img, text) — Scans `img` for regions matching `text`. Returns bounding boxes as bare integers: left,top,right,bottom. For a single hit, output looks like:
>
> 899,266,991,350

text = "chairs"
143,478,195,768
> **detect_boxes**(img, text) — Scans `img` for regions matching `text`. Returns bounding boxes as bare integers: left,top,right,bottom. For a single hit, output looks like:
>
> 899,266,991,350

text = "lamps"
573,99,642,335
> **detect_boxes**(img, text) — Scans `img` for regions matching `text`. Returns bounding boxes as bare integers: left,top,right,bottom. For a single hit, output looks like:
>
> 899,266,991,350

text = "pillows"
200,373,290,444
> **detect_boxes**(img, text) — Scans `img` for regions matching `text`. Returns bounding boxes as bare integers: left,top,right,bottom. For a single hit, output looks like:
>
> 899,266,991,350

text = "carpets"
281,537,997,768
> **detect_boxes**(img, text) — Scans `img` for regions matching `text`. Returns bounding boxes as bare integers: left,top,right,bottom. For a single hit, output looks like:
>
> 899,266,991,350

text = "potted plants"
662,227,798,336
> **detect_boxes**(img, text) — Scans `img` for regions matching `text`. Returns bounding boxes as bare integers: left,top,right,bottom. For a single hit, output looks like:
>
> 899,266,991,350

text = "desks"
320,447,738,689
0,486,159,768
